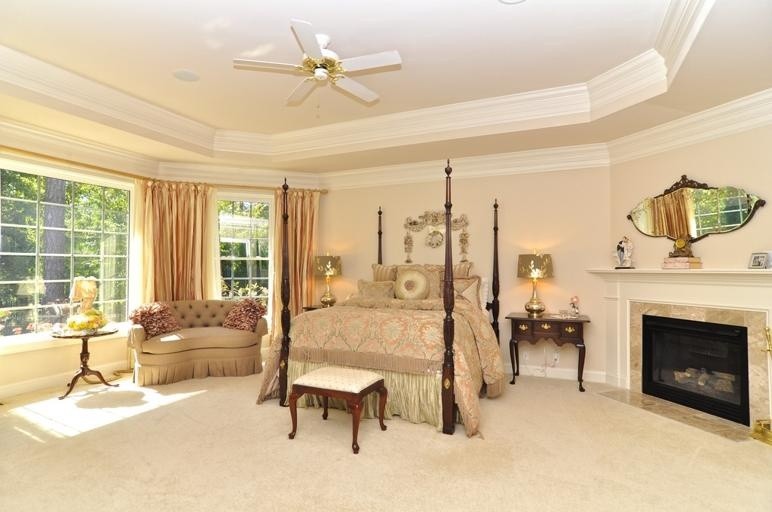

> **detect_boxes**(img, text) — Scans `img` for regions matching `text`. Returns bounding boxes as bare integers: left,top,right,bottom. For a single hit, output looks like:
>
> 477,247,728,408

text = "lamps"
517,253,555,318
314,255,342,308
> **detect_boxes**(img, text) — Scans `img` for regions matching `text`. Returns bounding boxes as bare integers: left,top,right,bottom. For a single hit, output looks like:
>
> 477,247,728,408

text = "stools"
288,366,388,454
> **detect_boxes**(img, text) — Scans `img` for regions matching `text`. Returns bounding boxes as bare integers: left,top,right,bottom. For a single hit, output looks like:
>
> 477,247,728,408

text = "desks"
504,312,591,392
52,327,119,400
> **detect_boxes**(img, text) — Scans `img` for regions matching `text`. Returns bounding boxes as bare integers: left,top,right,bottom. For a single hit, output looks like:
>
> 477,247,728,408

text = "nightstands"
303,304,320,311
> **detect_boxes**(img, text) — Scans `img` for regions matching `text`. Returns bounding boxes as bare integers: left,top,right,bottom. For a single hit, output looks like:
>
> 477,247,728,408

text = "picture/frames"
748,253,767,269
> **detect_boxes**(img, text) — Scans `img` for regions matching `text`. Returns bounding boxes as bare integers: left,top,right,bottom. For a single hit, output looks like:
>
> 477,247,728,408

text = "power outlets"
551,352,561,364
520,352,530,360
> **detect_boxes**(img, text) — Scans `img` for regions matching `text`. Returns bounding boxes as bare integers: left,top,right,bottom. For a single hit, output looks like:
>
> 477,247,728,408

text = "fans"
232,18,402,108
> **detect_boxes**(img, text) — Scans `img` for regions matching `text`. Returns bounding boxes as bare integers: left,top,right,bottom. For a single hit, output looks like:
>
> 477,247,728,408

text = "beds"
257,155,504,438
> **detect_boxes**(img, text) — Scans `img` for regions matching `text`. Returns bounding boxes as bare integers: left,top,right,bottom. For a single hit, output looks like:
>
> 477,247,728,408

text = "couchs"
132,299,268,386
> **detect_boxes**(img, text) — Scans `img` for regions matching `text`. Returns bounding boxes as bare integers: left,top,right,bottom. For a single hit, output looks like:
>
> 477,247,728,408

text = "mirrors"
627,175,766,257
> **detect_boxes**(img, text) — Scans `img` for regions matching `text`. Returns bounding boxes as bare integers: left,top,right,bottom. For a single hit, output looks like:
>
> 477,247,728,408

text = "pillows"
357,279,395,297
128,300,182,339
372,263,396,280
223,299,265,331
440,275,483,309
395,270,430,300
428,268,441,299
